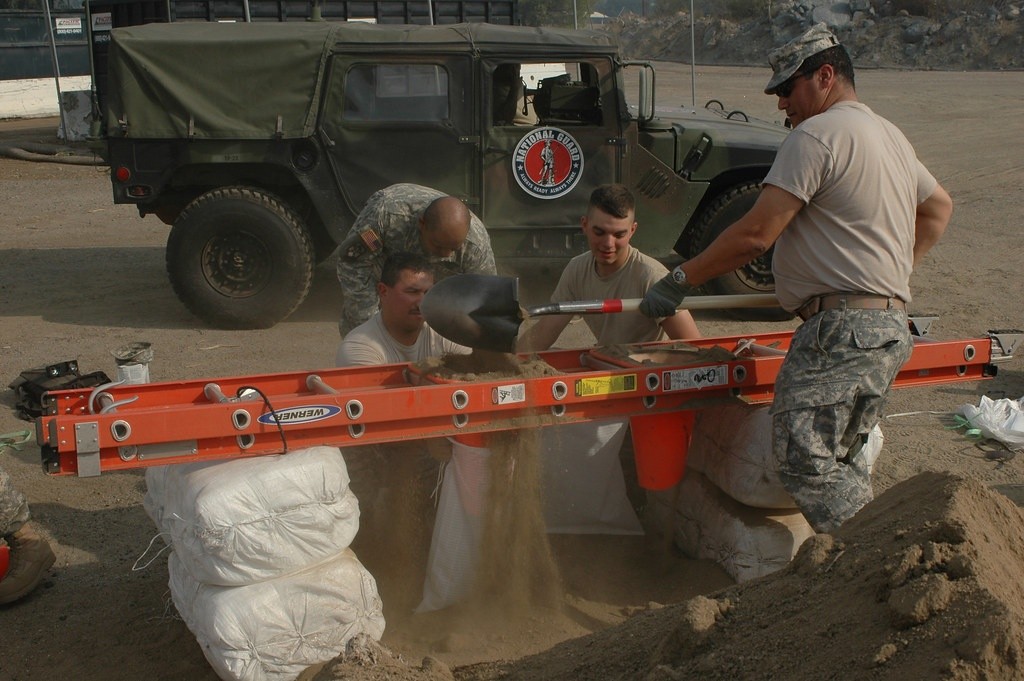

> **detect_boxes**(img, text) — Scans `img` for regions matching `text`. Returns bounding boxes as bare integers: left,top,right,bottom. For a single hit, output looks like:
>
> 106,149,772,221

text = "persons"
334,249,475,560
494,62,540,126
0,469,55,604
637,21,952,534
345,68,378,119
338,182,498,550
514,184,701,356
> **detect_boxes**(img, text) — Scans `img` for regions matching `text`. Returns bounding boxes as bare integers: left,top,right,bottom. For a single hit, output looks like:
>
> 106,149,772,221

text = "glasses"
774,69,810,98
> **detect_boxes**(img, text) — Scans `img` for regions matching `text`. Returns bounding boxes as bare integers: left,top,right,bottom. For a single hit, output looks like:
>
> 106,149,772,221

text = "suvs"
96,20,796,324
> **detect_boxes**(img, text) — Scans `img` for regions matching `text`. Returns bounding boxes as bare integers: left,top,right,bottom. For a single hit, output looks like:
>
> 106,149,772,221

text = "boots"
0,520,58,604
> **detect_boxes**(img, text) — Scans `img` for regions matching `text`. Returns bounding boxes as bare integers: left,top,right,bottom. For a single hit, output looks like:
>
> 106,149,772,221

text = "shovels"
416,273,782,356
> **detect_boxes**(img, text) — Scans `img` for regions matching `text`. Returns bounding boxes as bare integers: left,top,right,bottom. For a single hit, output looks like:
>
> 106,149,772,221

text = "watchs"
673,265,692,290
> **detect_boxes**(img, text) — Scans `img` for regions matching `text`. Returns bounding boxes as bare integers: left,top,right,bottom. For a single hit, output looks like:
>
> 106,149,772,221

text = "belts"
797,294,905,322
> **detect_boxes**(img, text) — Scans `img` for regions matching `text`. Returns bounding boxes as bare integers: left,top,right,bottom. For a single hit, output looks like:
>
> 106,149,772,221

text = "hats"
764,22,841,95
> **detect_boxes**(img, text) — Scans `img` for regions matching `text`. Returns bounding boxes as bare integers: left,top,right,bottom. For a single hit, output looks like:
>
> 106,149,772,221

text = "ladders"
34,314,1022,477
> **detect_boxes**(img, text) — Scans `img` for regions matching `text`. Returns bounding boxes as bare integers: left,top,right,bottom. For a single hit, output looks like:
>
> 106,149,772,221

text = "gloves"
640,273,686,317
